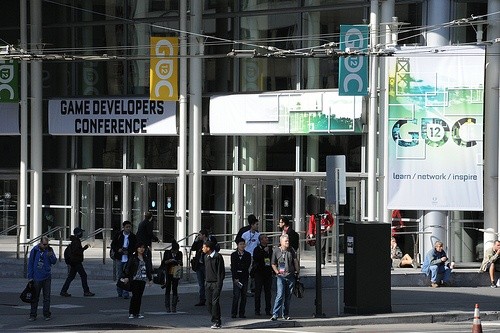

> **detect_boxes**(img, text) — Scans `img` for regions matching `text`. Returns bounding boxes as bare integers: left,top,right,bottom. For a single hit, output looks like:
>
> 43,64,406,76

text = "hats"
74,227,86,233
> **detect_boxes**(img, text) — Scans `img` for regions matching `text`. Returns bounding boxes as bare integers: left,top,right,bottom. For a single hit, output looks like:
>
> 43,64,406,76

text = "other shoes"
137,314,144,318
129,314,134,318
211,323,221,328
118,293,122,297
247,292,254,297
194,303,205,306
239,315,247,318
432,283,438,287
84,291,95,296
29,317,35,321
46,316,50,320
491,283,497,288
255,312,261,316
266,313,272,316
270,315,277,321
166,307,171,312
282,315,291,320
124,294,129,298
60,291,72,297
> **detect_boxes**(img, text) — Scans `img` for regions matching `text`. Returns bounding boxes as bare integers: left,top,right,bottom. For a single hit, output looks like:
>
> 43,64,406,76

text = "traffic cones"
470,304,484,333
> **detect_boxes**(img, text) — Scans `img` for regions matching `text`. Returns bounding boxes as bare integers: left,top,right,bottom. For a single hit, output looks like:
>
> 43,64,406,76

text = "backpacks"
110,233,121,260
64,242,73,265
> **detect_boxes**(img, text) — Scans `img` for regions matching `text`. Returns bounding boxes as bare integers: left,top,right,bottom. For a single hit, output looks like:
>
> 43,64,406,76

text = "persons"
309,210,334,269
279,216,299,252
202,241,226,328
46,210,54,238
230,238,251,318
162,243,183,313
421,241,454,287
192,229,220,306
111,212,160,298
391,210,417,268
235,214,261,297
480,240,500,288
271,234,299,320
27,236,57,321
60,227,95,297
252,234,273,316
125,241,153,319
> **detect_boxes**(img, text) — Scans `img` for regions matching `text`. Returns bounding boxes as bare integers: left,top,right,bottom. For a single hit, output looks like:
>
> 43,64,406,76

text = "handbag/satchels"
116,264,132,291
20,281,33,303
191,257,197,272
294,281,305,298
